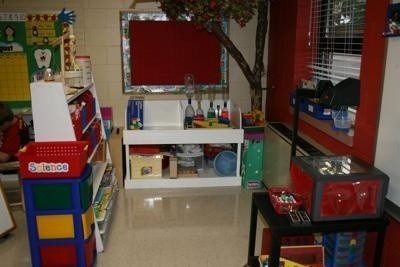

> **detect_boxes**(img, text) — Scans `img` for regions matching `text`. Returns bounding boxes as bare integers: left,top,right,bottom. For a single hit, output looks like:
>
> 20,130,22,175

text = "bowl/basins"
213,150,237,176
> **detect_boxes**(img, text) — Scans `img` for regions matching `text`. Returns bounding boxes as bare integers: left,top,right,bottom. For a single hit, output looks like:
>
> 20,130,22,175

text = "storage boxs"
129,153,163,179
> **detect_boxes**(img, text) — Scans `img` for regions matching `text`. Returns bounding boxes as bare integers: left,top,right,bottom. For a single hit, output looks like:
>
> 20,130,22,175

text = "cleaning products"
185,99,229,129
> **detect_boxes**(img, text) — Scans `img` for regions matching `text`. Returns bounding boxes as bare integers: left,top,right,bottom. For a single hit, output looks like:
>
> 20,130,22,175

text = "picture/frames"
0,189,17,237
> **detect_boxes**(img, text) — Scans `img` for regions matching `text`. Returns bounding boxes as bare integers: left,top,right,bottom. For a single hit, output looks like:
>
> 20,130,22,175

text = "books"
92,163,118,243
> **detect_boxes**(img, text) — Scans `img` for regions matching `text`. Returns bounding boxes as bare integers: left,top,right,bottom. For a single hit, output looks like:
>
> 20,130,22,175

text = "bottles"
207,101,215,125
70,58,83,85
222,102,229,119
185,98,195,125
196,101,203,126
44,68,55,82
216,105,221,120
18,112,23,128
334,105,352,129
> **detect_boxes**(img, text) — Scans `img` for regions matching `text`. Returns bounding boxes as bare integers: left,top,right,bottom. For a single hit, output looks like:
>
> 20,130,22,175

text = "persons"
0,107,29,175
4,25,15,43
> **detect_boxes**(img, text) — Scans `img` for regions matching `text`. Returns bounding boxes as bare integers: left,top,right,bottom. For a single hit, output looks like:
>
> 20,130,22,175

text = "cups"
184,74,194,90
300,78,316,88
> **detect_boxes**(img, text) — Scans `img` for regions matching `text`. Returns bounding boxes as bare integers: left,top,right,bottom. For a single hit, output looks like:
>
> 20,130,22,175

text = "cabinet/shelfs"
123,99,245,189
248,192,389,267
30,71,118,253
23,164,97,267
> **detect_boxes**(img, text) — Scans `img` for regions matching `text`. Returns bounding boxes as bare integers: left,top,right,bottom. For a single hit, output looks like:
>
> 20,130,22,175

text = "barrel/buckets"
74,55,92,86
